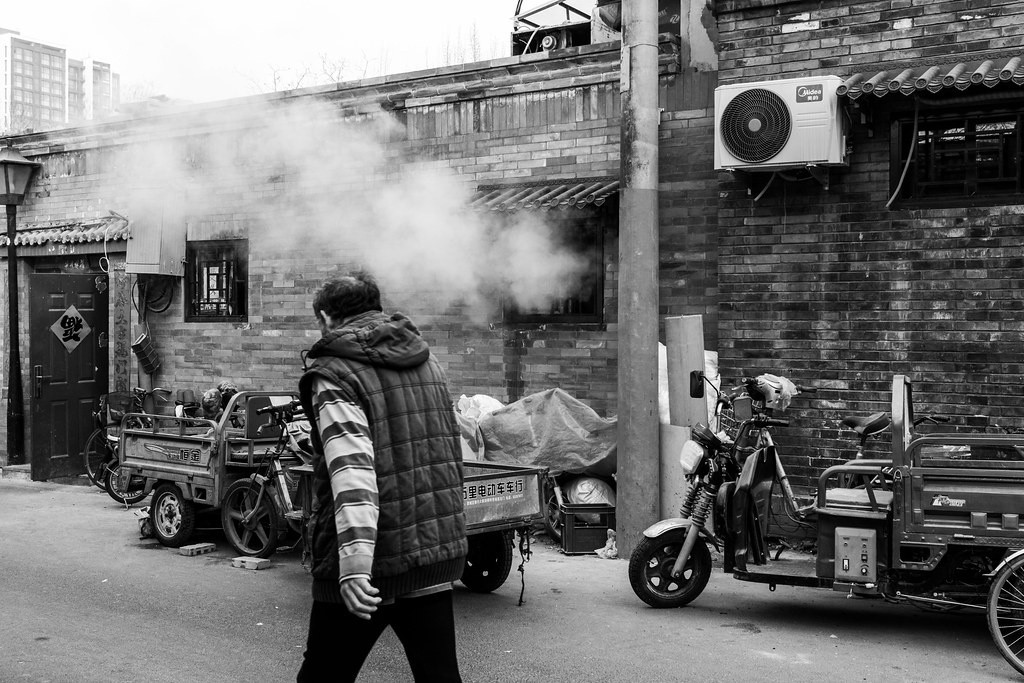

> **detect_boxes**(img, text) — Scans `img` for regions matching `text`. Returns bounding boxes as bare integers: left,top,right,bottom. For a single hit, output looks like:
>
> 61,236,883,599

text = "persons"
295,270,463,683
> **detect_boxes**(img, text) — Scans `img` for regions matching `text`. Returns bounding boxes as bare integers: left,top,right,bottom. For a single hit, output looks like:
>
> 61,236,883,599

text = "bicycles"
733,375,893,527
981,543,1024,674
85,384,201,489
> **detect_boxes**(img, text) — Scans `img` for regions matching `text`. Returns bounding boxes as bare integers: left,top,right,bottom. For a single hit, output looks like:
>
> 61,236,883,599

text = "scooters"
533,460,574,540
102,449,157,508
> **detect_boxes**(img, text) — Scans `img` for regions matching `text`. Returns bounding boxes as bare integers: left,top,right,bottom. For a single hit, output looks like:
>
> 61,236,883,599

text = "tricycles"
221,397,551,596
120,388,309,548
627,366,1024,613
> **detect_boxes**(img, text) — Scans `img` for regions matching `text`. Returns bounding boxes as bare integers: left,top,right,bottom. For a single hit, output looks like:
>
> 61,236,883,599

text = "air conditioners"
714,74,851,173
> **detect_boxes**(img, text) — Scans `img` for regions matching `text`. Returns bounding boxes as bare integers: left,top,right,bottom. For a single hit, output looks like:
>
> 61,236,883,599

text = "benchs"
817,375,915,519
223,394,307,467
